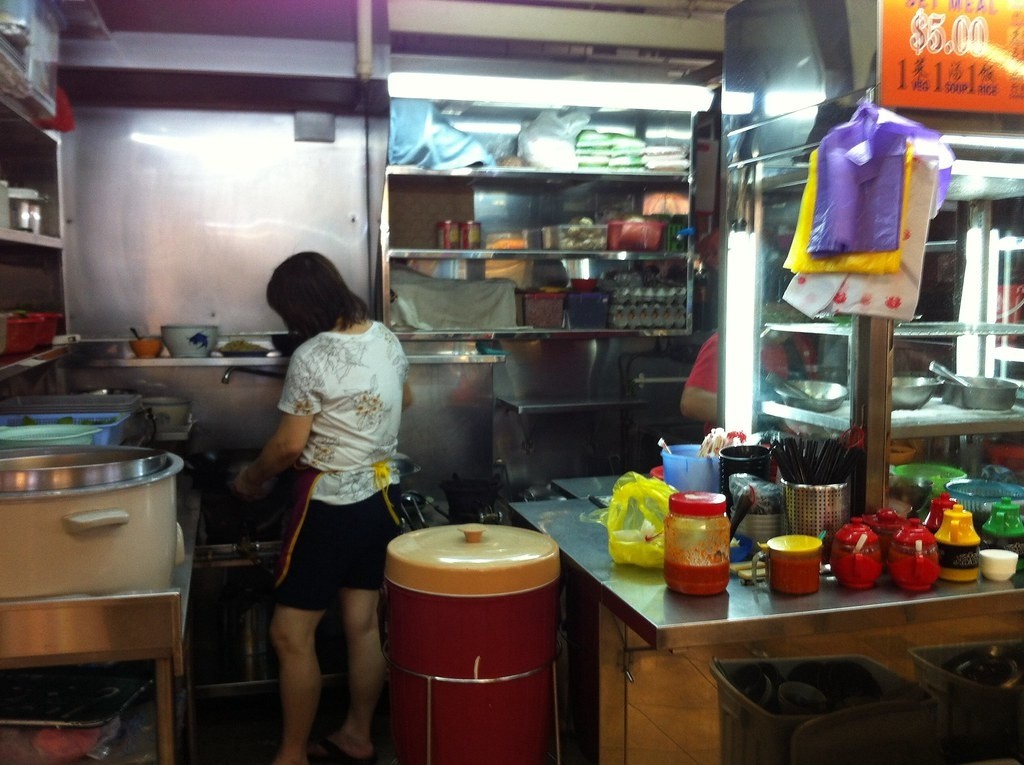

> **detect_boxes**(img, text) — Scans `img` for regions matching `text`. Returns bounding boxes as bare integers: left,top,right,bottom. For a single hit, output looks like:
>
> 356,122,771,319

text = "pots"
0,446,185,599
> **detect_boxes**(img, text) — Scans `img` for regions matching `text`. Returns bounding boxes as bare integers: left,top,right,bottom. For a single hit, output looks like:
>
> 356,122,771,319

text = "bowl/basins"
6,313,62,353
936,375,1018,410
271,334,307,356
161,325,218,358
570,278,599,290
773,379,848,413
141,396,192,433
890,376,936,412
130,339,162,359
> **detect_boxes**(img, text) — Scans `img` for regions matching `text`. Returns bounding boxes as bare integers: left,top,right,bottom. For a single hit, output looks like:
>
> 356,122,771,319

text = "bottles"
766,533,822,596
664,490,731,596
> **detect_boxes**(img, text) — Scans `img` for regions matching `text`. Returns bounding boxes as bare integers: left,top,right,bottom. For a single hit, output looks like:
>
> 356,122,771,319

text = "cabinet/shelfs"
719,87,1024,518
0,97,71,375
380,165,694,340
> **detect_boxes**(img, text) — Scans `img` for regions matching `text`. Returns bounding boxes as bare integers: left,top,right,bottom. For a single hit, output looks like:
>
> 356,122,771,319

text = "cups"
717,444,771,522
979,548,1019,581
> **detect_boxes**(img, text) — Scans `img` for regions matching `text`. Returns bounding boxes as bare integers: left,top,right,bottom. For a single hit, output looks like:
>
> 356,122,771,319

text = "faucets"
221,364,283,384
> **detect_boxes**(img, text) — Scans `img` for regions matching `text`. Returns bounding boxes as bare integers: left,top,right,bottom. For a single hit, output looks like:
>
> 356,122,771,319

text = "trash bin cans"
909,640,1024,765
708,656,939,764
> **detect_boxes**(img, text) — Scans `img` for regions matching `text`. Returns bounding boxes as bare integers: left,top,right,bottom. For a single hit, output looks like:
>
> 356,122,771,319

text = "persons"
679,273,818,444
204,252,414,765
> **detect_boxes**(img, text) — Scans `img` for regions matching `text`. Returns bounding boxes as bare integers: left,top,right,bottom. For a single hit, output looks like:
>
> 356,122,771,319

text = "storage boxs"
542,224,609,251
524,293,566,328
606,219,666,251
563,291,611,330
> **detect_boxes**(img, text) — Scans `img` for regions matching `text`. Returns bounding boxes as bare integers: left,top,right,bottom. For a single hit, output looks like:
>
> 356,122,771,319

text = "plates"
220,350,271,357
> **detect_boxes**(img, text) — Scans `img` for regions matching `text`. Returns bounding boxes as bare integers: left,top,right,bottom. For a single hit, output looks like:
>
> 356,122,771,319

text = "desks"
0,494,202,765
511,493,1024,765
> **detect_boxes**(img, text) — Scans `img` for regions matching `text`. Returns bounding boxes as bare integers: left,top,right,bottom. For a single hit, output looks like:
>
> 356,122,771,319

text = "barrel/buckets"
383,521,561,765
660,444,720,494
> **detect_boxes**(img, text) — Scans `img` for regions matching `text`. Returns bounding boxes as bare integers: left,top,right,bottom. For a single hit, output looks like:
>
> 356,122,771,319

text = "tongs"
765,371,813,400
929,360,971,389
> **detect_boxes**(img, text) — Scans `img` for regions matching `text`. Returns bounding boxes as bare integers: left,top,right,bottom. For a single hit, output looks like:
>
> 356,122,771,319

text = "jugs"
829,493,1024,592
